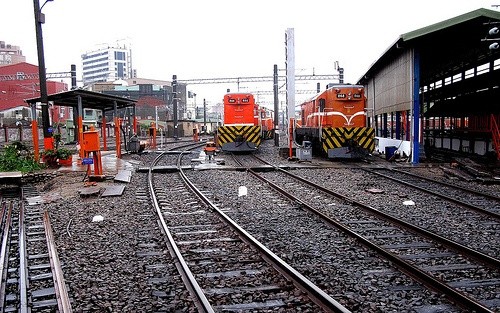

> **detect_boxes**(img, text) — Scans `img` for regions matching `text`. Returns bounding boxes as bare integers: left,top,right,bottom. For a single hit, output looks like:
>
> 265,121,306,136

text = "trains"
295,84,376,158
217,91,273,150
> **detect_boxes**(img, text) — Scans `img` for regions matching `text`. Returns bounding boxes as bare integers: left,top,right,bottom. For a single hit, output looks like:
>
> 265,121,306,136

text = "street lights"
32,0,54,137
